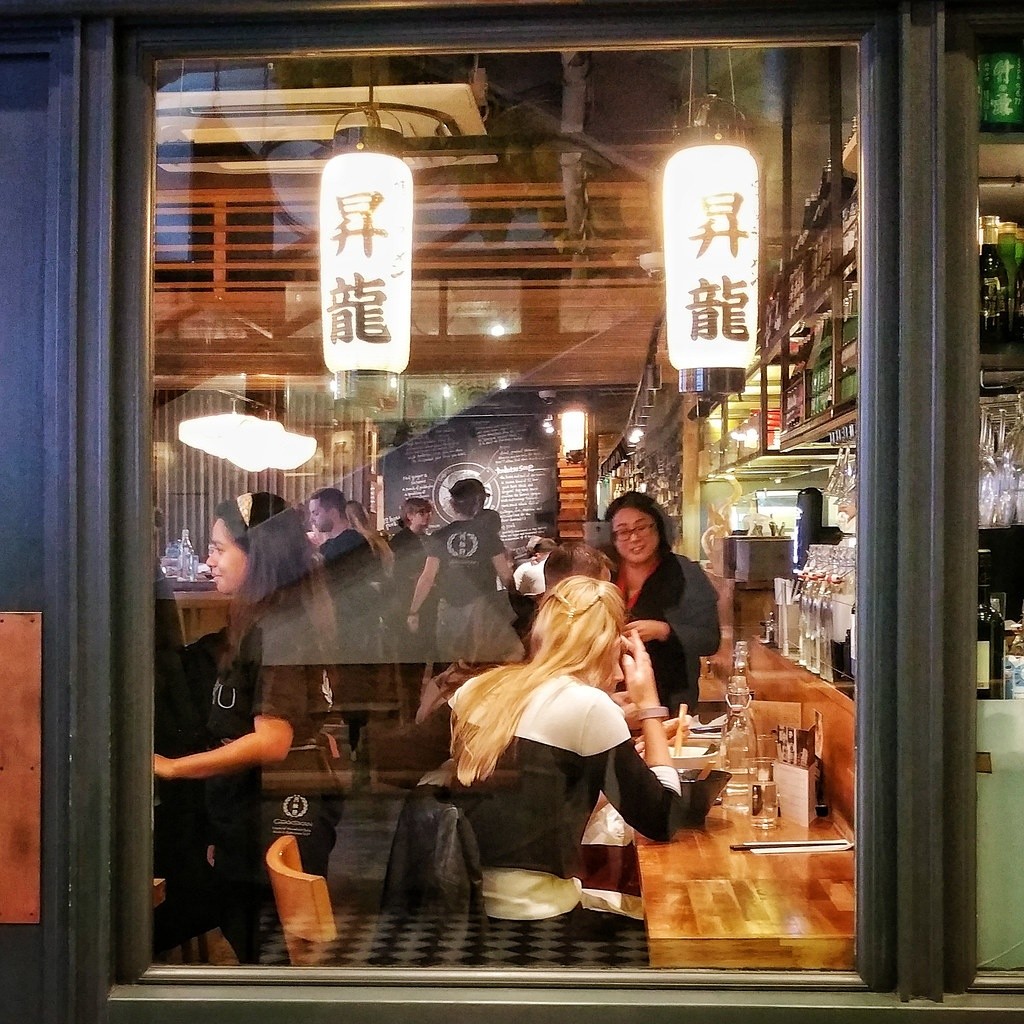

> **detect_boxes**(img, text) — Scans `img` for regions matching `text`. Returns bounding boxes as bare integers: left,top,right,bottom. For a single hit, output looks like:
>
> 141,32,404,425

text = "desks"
631,636,856,970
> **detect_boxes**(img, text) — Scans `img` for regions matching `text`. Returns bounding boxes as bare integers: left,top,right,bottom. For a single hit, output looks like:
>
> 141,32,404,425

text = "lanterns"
320,124,415,406
661,122,760,393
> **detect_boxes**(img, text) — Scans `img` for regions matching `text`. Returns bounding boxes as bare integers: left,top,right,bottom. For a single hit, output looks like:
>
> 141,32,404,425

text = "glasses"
613,523,654,540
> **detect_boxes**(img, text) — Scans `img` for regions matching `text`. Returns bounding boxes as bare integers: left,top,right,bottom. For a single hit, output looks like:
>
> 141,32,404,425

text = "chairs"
411,785,493,967
267,834,339,968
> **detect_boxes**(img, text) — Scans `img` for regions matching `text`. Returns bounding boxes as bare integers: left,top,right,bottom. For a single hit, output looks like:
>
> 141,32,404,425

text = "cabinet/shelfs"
697,34,1024,489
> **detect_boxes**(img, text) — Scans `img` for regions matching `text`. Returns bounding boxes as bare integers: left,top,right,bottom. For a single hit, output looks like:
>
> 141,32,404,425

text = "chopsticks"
775,578,802,605
730,843,847,851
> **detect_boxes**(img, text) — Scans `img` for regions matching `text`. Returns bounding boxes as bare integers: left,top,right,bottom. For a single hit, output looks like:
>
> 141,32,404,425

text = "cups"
749,781,777,830
748,735,776,760
748,758,776,783
194,555,199,574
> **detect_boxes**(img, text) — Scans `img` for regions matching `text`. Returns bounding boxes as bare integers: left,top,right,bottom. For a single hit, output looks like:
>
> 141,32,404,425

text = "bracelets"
634,705,669,722
406,610,418,617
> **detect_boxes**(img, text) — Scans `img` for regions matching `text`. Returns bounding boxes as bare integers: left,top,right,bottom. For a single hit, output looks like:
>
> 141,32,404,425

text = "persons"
152,477,723,972
837,504,858,522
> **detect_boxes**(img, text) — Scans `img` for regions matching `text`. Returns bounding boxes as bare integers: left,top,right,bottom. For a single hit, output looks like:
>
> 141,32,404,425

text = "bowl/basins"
668,769,733,826
666,737,727,769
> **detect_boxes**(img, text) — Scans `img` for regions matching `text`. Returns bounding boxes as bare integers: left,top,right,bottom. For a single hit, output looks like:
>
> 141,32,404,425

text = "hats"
449,479,491,500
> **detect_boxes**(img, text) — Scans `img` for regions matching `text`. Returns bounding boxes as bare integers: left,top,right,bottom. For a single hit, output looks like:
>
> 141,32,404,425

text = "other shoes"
219,908,260,965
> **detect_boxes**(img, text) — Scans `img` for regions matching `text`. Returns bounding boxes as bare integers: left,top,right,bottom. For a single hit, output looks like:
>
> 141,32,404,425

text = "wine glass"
978,402,1024,530
817,440,860,520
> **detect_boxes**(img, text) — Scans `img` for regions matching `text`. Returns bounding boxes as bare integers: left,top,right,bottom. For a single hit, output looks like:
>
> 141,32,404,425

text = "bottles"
976,548,1005,700
725,641,759,797
177,529,195,582
798,576,834,682
787,282,858,429
795,158,860,230
980,215,1024,353
614,483,620,498
